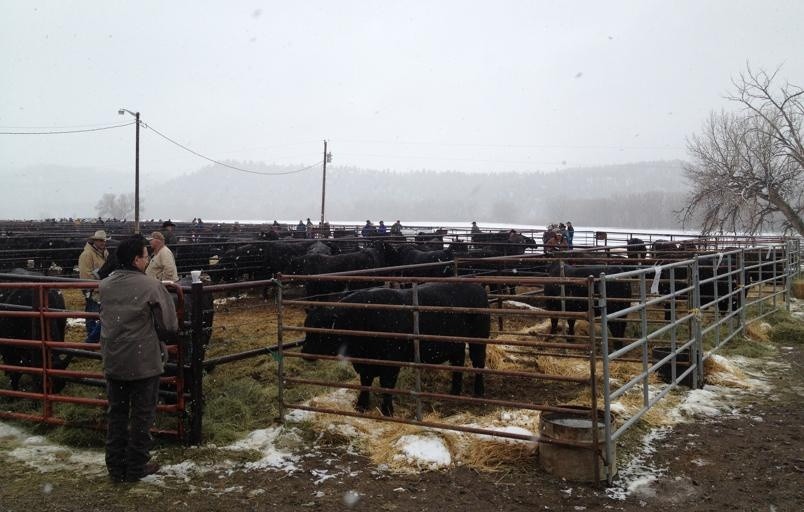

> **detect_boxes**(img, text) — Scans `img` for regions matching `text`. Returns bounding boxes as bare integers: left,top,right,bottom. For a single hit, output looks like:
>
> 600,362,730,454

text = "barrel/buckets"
538,406,618,483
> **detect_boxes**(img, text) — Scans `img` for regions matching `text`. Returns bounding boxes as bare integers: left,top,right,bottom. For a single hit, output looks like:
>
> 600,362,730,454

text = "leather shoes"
139,464,159,477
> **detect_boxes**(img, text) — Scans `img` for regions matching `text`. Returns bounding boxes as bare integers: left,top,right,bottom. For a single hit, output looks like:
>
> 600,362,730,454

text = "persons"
541,221,574,252
469,222,480,249
273,220,280,226
192,217,202,225
296,219,306,231
306,218,315,239
147,231,178,288
160,221,178,258
366,220,402,232
45,215,127,223
78,229,112,343
98,232,178,485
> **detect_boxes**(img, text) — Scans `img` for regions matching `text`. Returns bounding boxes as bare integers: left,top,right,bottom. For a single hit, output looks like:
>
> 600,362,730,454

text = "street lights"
118,106,142,236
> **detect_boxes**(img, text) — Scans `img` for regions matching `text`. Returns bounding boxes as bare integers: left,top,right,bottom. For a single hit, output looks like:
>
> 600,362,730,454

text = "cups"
190,271,202,283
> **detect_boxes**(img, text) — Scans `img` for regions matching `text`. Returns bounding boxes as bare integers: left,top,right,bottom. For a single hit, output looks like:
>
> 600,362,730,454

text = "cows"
299,280,491,420
0,268,74,396
544,263,631,351
152,274,214,403
0,218,537,312
660,261,750,323
626,237,646,261
652,240,794,283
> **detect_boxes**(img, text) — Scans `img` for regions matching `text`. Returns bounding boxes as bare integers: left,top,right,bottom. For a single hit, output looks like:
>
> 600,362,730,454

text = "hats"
472,222,476,224
559,224,566,228
556,230,562,236
89,231,111,241
146,232,164,241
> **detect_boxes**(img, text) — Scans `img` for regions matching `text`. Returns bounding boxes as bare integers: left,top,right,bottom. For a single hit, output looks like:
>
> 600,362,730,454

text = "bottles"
27,260,34,269
